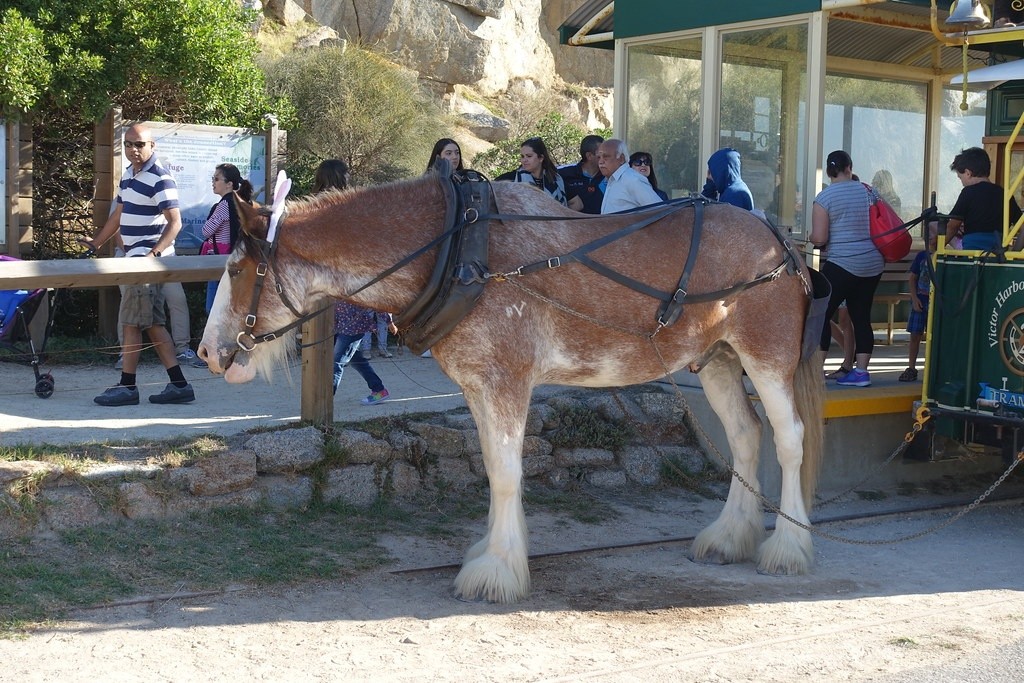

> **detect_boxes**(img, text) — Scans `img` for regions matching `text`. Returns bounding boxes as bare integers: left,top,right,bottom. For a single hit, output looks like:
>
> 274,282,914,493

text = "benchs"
868,253,918,344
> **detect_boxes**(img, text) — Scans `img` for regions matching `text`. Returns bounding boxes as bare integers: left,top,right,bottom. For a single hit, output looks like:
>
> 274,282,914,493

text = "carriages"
195,0,1024,604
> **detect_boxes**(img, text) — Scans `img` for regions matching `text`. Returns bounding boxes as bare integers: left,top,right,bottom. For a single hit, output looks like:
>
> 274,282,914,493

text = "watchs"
150,248,161,257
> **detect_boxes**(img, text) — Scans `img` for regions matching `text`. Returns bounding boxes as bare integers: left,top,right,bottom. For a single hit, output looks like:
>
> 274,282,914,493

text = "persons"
108,195,207,370
597,138,665,214
898,213,937,381
417,138,463,358
944,147,1022,252
557,135,605,214
808,149,885,387
629,151,668,202
193,164,399,404
79,123,196,407
494,135,569,211
703,147,754,211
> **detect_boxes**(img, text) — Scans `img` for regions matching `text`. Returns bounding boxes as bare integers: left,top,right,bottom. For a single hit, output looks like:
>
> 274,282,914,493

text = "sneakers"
149,383,196,404
828,366,849,379
114,353,122,369
361,388,390,404
176,348,208,368
836,369,872,386
94,383,139,406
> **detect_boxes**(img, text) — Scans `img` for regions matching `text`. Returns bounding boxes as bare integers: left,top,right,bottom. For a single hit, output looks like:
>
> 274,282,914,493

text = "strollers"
0,237,96,397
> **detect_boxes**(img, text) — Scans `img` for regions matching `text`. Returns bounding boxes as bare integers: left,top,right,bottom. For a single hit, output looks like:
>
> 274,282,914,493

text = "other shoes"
363,350,372,359
379,349,392,357
421,349,431,357
899,368,918,381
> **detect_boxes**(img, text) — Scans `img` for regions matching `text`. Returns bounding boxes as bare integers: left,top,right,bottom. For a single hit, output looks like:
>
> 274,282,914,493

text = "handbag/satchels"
862,183,912,262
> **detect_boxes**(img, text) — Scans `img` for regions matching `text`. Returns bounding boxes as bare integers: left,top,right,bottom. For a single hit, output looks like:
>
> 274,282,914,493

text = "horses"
198,172,825,605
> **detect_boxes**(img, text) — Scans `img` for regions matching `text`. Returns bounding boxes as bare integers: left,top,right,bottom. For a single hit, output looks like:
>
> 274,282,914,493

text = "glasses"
532,136,542,145
212,177,226,181
124,140,151,148
633,158,652,166
